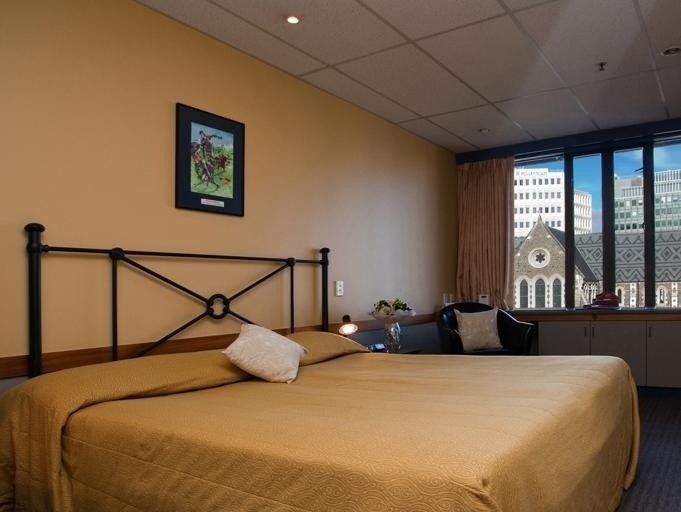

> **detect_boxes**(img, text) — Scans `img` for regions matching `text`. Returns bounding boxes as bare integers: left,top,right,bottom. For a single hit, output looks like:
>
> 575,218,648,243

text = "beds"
14,222,637,511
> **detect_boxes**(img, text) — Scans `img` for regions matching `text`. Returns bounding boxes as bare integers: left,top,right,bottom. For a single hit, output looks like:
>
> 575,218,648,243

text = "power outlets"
336,280,345,296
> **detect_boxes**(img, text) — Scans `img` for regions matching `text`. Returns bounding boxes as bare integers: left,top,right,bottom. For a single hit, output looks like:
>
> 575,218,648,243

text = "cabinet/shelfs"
538,319,647,388
646,321,681,388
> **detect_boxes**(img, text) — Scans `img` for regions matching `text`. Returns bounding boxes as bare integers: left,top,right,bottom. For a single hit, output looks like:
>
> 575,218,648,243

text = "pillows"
223,323,311,383
454,306,503,353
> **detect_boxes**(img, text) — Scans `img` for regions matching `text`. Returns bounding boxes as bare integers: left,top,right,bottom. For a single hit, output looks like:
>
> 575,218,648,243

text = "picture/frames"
175,102,246,218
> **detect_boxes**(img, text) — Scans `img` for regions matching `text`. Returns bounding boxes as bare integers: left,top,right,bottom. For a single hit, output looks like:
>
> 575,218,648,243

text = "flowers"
370,299,417,320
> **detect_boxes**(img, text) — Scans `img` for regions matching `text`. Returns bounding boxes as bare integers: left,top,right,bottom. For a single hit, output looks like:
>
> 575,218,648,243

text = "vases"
383,322,402,353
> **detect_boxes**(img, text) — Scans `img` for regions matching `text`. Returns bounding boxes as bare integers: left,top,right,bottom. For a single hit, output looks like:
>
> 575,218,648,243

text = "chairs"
437,301,536,356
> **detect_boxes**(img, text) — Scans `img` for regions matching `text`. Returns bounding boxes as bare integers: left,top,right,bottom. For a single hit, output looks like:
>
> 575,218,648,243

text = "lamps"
338,315,359,337
